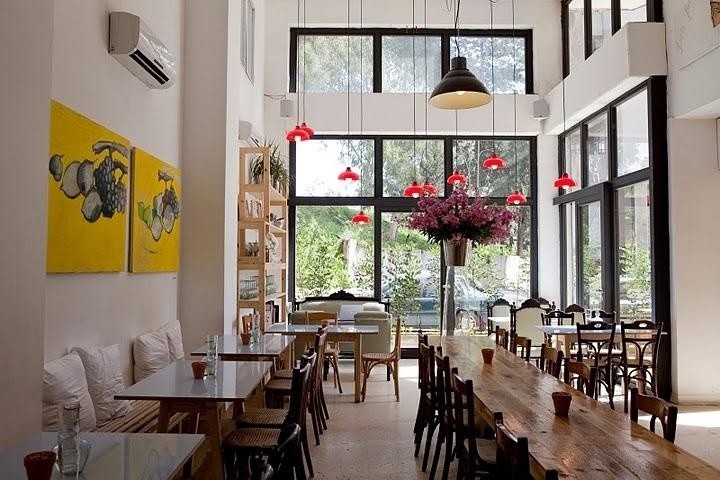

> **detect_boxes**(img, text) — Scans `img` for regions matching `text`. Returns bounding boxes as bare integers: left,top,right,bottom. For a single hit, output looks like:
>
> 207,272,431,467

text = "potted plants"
251,136,293,191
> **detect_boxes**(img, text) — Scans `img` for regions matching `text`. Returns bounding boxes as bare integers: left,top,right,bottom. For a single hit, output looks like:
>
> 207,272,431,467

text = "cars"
382,275,494,331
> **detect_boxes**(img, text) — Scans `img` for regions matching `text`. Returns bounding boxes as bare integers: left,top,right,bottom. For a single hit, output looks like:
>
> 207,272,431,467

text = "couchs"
299,302,366,352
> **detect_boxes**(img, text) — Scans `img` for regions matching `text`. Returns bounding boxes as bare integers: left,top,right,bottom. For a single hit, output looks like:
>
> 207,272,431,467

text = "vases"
443,238,469,266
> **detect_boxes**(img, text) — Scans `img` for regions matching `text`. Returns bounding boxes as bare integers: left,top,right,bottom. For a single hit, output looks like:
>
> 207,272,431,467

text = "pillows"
74,343,132,422
336,305,365,320
44,351,97,433
130,327,171,384
161,319,185,361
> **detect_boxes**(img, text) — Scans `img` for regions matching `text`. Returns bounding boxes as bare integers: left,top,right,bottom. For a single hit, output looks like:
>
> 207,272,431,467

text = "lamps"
301,1,314,137
447,110,467,186
421,0,438,198
338,0,360,181
429,0,493,110
287,0,309,141
351,0,372,224
555,0,577,189
404,0,424,199
506,0,528,204
484,1,506,170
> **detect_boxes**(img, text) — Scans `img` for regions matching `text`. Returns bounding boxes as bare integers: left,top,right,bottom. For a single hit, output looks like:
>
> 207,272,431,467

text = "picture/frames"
129,144,183,272
46,97,131,274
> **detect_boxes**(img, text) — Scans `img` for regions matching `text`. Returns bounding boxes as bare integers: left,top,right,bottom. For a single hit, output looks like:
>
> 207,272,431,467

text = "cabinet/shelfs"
239,146,288,333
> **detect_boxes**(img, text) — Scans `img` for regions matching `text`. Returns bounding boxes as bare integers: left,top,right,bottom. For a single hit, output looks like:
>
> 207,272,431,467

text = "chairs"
264,333,328,445
540,312,589,386
537,297,556,313
545,469,558,480
451,366,498,480
290,311,327,360
414,339,470,473
242,313,253,333
590,310,624,398
417,330,451,391
512,333,531,363
495,325,509,350
613,321,664,413
250,423,301,480
485,298,516,337
353,311,394,382
219,363,310,479
565,304,584,325
510,298,552,369
303,311,343,394
273,326,330,430
574,323,616,412
494,411,535,480
362,302,385,312
540,344,563,379
238,352,319,478
362,315,401,402
429,341,479,480
631,388,678,444
564,357,597,398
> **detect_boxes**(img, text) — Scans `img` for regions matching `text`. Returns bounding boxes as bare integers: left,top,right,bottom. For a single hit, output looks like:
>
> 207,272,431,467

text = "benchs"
90,400,192,436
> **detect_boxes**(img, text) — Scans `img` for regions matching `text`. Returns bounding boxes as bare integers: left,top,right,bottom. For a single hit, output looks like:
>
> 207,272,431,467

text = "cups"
203,333,220,377
251,313,261,345
482,348,495,364
240,333,251,345
552,391,572,416
22,452,58,480
321,319,329,329
192,361,208,381
52,400,92,476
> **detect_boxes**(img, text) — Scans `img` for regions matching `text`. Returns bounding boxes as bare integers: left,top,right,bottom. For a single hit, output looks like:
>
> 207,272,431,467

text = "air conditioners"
109,13,175,89
237,120,264,148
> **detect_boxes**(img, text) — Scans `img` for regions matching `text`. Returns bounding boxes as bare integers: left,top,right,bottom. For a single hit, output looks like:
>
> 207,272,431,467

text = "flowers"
397,184,517,245
